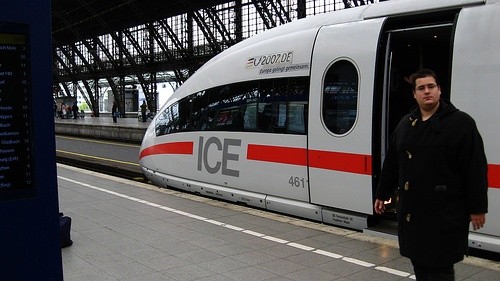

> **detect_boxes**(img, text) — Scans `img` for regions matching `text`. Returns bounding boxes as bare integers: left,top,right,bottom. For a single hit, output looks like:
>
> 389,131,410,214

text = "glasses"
415,84,438,91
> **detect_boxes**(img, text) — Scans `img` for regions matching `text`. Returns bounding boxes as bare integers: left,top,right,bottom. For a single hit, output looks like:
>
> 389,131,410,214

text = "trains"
139,1,500,254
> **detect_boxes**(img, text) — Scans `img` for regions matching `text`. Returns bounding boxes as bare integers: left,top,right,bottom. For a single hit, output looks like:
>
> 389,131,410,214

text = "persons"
54,100,88,118
373,65,488,281
111,101,118,122
140,99,147,121
